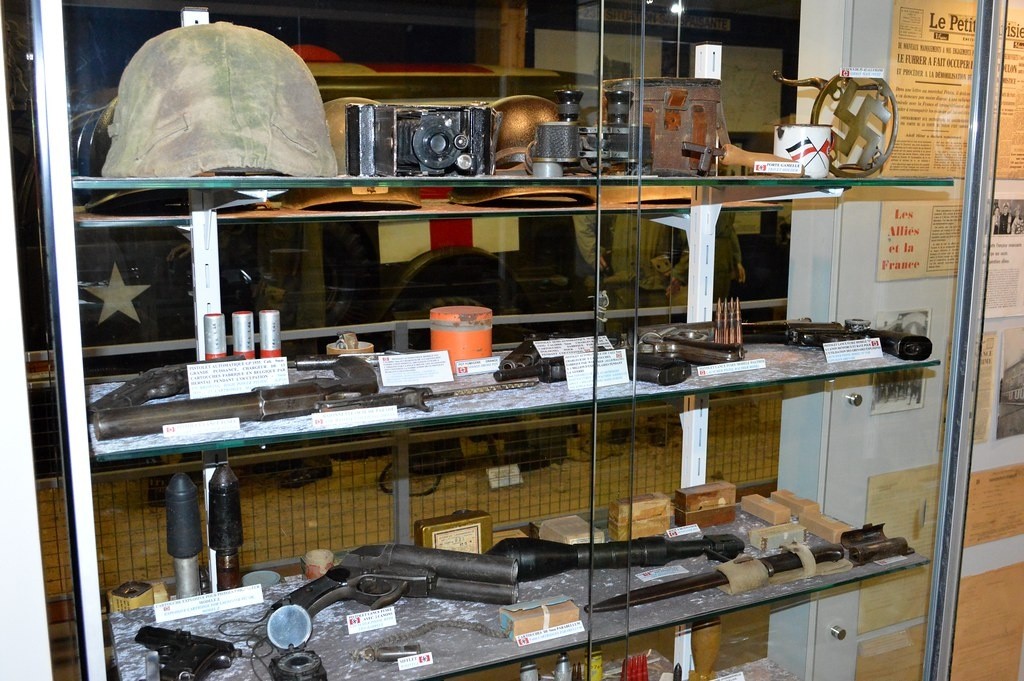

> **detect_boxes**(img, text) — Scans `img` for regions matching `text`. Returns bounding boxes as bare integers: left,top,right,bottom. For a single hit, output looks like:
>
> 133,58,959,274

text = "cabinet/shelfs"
31,0,1007,679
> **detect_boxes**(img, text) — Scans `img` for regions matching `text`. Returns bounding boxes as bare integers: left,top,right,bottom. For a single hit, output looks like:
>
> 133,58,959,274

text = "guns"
270,544,518,617
494,316,932,382
134,625,241,681
87,354,380,439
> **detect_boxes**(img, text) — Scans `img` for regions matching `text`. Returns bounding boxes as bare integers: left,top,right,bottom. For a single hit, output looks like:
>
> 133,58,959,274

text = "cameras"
345,102,494,176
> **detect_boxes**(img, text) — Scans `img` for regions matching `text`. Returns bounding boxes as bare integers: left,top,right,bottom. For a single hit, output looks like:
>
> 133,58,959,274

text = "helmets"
101,23,337,178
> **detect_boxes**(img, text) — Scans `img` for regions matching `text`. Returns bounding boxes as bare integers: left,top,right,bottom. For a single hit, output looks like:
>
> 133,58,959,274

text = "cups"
429,306,492,374
772,123,833,179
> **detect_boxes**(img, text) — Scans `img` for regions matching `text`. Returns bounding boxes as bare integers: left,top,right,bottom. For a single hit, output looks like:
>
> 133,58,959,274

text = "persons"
519,213,745,335
990,202,1023,234
